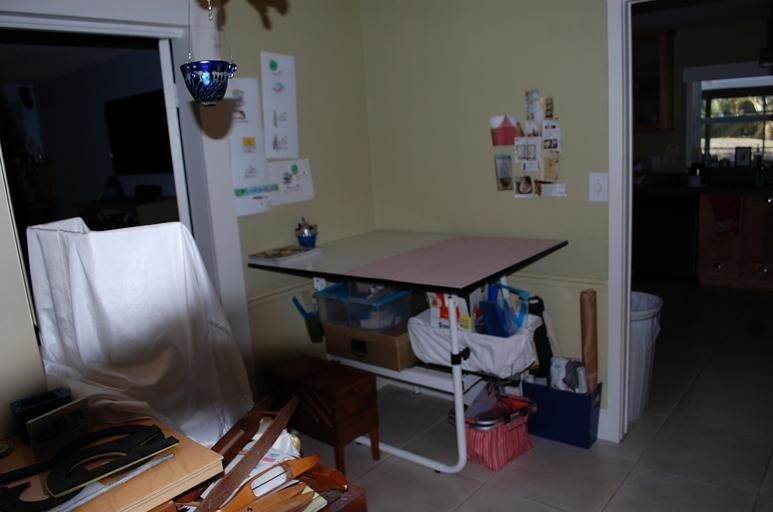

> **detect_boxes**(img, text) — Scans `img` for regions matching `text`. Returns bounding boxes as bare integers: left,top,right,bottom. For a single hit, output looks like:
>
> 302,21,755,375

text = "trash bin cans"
629,291,663,421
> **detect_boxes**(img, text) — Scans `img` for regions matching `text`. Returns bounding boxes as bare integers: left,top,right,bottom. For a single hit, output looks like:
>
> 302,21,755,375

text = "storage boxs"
450,372,601,467
315,282,543,370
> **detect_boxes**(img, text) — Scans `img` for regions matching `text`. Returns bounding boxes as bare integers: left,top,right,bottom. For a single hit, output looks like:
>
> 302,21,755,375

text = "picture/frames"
631,30,673,132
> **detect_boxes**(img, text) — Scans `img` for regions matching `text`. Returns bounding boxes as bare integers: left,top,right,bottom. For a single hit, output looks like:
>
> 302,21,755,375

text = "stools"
269,357,381,474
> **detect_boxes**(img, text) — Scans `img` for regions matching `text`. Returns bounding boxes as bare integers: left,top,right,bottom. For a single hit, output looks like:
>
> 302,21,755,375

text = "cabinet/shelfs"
695,194,773,291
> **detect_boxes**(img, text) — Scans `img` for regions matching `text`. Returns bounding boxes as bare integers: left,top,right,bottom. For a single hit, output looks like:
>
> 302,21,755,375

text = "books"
247,244,321,266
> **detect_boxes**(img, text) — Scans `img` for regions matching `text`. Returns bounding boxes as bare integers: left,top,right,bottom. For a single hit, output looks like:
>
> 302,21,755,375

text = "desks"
0,393,226,512
247,229,570,476
